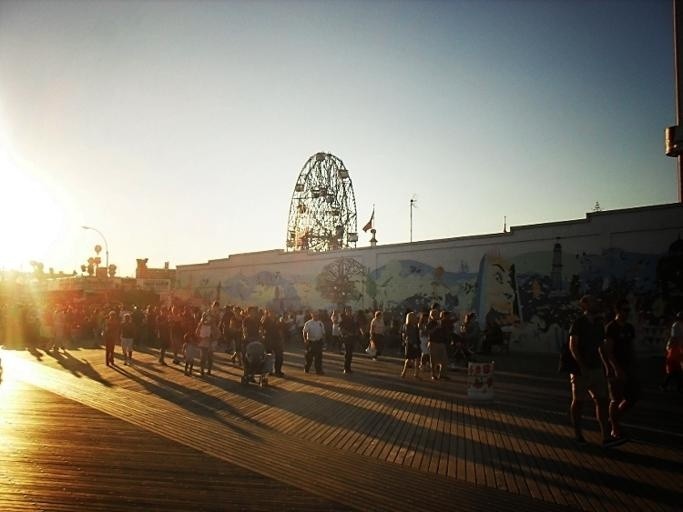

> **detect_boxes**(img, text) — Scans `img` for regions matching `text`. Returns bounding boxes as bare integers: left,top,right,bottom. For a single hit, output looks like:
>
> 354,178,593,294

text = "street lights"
81,224,109,277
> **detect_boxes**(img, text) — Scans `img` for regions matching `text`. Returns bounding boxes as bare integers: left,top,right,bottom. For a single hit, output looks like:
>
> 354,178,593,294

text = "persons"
658,337,683,394
601,298,644,439
566,294,627,448
667,311,683,393
0,294,477,389
